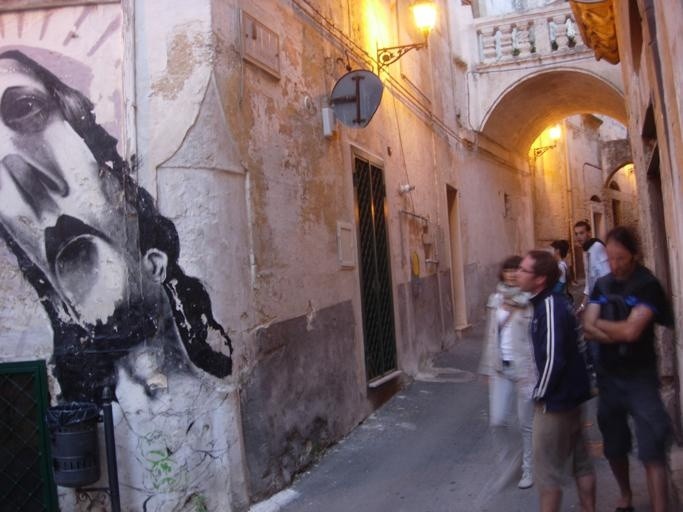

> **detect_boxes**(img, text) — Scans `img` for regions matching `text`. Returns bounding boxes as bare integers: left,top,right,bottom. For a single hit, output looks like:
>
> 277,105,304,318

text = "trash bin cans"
44,402,99,486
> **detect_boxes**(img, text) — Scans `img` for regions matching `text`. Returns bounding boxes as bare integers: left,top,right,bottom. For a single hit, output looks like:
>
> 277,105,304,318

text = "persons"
513,252,597,510
574,220,611,376
577,227,677,510
0,49,236,405
545,241,571,296
476,252,538,490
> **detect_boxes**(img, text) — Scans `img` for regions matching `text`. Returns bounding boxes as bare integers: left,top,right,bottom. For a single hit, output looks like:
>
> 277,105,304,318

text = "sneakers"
518,476,533,490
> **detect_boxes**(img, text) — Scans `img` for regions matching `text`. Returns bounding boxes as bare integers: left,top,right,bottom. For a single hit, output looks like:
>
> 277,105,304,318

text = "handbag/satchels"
588,295,630,371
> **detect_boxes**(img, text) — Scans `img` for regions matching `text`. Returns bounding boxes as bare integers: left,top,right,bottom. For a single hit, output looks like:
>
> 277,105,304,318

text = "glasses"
519,265,535,276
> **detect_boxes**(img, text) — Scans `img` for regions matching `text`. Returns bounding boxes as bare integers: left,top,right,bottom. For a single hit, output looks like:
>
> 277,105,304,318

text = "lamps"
377,2,439,77
534,123,561,160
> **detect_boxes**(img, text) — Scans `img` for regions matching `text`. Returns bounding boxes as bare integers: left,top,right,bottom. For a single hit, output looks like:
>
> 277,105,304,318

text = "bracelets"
578,303,586,311
